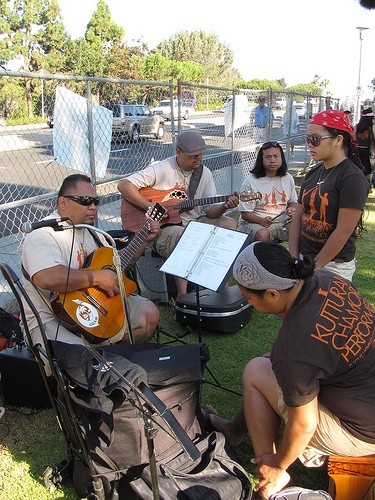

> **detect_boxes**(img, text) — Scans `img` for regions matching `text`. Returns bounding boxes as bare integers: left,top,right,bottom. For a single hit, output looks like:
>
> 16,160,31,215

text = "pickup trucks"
101,103,166,143
151,99,193,121
270,97,296,109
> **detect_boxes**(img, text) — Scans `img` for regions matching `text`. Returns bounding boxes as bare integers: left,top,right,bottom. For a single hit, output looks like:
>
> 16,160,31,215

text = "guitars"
50,202,168,345
121,186,263,234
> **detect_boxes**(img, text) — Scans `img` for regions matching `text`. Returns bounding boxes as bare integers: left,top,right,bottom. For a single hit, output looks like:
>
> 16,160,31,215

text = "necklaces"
316,164,337,184
180,167,192,185
71,228,87,253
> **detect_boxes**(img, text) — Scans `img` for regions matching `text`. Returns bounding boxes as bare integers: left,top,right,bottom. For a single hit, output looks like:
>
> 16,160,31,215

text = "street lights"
39,68,45,122
206,78,209,107
356,26,370,124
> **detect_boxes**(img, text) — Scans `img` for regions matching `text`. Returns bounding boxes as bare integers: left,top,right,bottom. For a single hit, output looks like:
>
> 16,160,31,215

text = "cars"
292,102,319,119
47,116,54,129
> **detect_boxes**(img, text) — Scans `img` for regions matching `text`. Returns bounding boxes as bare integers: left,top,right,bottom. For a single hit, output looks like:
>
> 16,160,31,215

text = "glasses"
60,195,101,207
305,134,334,147
262,142,280,149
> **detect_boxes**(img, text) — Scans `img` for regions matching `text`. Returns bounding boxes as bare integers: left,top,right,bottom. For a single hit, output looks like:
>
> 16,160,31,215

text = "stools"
326,453,375,500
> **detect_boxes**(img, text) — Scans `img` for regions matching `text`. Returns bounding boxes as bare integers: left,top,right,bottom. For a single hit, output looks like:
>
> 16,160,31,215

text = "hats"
257,96,265,100
176,131,207,153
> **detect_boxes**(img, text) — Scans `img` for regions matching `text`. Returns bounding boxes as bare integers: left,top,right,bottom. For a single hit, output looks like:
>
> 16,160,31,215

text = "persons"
209,241,375,500
21,174,160,390
249,98,274,156
237,142,297,241
117,131,239,299
289,110,370,281
281,105,299,155
346,109,375,175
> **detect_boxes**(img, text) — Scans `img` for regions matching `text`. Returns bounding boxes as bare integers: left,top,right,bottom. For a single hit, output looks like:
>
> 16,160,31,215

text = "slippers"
200,403,243,450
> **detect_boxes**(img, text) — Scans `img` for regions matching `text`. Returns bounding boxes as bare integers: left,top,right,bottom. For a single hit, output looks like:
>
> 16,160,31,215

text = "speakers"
136,248,178,305
0,341,57,409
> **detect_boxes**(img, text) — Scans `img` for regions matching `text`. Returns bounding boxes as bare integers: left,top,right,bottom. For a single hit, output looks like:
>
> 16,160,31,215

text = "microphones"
20,217,71,232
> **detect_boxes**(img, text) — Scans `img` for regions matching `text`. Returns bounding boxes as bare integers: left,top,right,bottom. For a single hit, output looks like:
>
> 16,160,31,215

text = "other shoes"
291,152,295,157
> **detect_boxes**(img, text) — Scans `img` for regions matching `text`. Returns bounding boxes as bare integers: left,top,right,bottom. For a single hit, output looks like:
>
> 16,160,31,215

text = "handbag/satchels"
51,335,210,499
130,431,254,500
